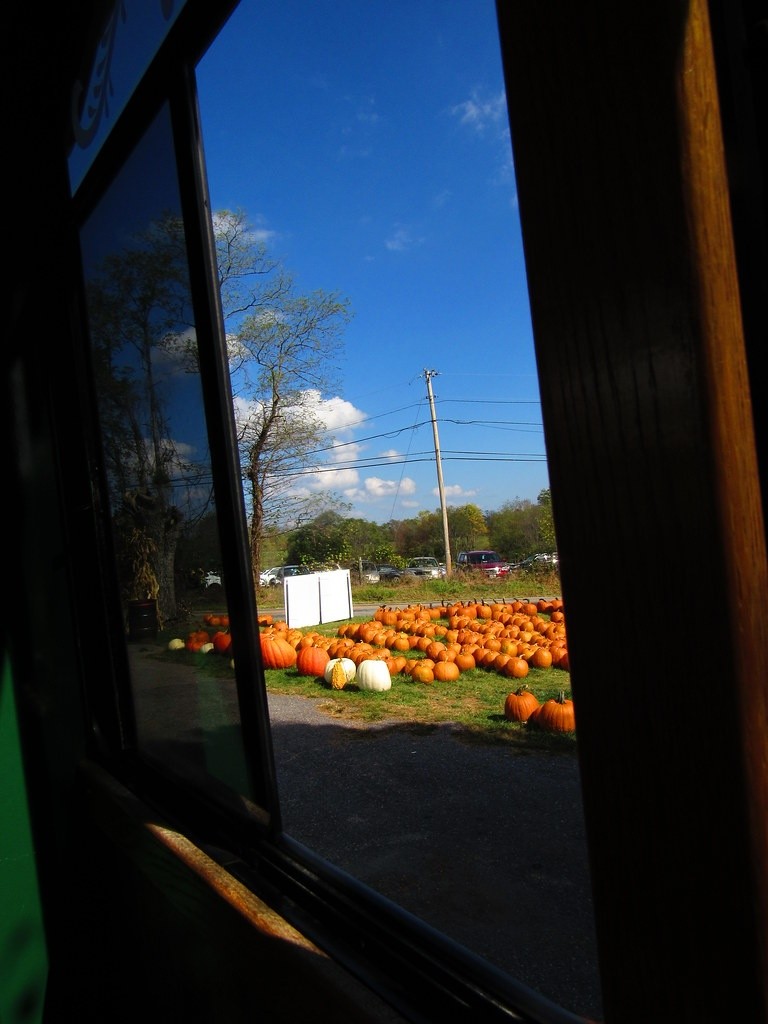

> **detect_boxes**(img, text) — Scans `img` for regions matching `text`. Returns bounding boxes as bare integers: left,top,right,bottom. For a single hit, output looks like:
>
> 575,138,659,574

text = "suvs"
455,551,512,581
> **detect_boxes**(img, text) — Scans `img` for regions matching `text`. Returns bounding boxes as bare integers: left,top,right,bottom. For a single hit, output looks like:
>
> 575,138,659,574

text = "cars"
406,556,442,581
512,554,559,572
202,570,222,590
353,560,380,585
375,564,405,584
438,563,448,578
259,566,312,587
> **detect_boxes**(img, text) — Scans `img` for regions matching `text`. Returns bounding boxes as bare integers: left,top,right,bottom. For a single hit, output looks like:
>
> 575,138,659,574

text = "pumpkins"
166,596,576,734
